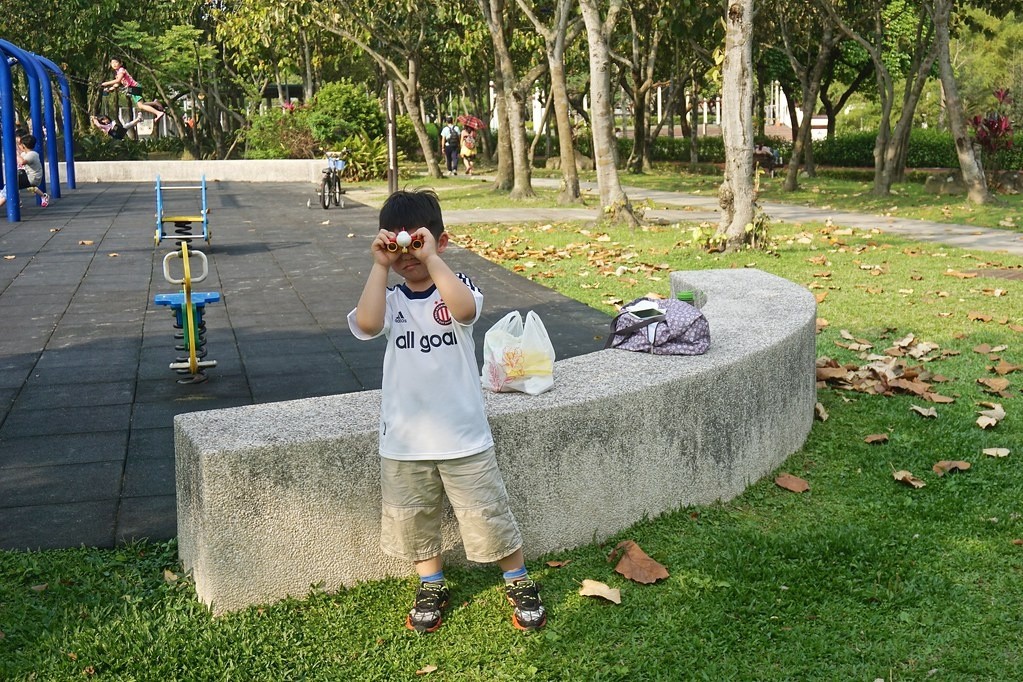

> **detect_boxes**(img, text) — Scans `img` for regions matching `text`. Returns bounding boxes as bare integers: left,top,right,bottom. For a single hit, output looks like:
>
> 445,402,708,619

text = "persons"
102,56,164,122
346,189,547,632
0,127,50,208
441,115,461,175
753,142,775,176
90,111,144,140
460,125,477,175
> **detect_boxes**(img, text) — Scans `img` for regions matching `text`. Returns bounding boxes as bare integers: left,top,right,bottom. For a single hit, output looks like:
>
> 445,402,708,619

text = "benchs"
172,268,818,619
751,153,780,180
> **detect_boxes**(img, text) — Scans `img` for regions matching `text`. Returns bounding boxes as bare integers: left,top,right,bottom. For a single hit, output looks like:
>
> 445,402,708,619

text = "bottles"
676,288,704,307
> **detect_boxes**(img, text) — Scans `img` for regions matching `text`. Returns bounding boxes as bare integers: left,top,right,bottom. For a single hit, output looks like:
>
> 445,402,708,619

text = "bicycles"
314,146,354,210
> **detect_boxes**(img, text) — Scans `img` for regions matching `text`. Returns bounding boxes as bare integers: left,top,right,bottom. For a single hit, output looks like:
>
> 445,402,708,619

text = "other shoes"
448,172,451,175
454,171,457,175
466,166,472,174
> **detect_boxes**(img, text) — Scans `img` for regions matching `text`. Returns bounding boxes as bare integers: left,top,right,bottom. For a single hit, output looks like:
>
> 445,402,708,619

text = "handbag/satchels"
603,294,710,356
480,309,557,395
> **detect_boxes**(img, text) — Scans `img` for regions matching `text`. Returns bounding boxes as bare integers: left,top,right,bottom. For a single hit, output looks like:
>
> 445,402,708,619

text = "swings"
44,67,144,97
10,56,128,141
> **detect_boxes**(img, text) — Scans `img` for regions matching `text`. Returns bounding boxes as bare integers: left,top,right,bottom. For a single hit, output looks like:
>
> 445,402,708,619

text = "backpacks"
446,124,460,148
464,130,475,149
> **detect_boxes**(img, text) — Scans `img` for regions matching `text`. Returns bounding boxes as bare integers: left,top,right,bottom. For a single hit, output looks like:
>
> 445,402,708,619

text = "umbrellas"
457,114,487,129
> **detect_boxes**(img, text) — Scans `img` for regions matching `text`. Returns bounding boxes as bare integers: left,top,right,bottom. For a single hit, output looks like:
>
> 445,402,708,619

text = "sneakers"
505,579,546,632
407,580,449,633
